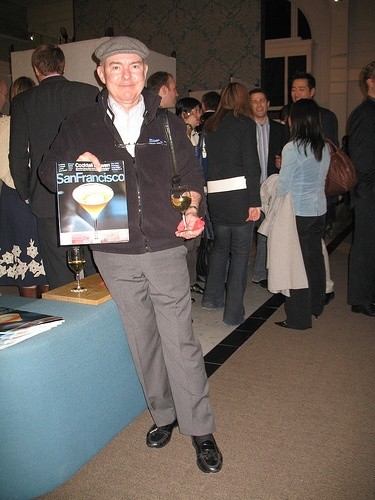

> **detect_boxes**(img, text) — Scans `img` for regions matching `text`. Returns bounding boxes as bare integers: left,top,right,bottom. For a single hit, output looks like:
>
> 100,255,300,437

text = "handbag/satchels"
323,135,358,197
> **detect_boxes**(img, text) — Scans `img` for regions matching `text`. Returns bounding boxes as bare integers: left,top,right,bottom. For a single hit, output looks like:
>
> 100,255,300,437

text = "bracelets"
189,206,198,211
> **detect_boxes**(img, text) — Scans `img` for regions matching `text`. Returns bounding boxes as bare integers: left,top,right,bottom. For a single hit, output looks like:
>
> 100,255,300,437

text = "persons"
0,44,374,330
38,35,224,474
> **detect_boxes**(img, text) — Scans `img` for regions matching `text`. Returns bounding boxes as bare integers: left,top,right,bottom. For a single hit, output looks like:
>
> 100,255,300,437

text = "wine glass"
71,182,114,230
169,185,192,234
66,246,90,293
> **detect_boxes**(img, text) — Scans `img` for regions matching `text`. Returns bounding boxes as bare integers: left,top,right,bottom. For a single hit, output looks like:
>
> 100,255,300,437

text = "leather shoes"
191,298,195,303
190,283,205,294
191,433,223,473
146,418,177,448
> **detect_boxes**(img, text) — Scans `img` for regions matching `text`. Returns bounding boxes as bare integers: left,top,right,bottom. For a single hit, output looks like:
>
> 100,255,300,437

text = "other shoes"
351,303,375,317
274,320,312,330
312,313,319,320
324,291,334,305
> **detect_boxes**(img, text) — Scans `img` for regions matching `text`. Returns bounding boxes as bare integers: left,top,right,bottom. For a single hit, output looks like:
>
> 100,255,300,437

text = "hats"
95,35,151,62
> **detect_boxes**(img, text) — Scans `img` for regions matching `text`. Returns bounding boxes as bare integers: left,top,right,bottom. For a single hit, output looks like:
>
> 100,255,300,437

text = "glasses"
111,114,150,150
